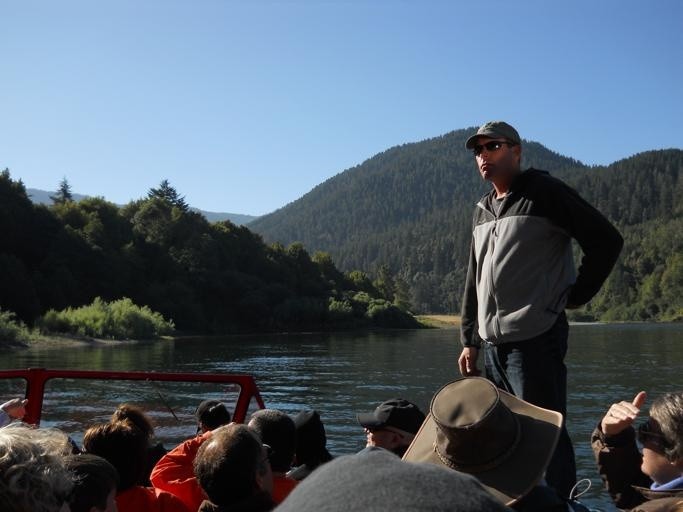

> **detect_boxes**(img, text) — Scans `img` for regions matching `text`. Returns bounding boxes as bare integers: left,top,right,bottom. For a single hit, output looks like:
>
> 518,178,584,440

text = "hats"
293,407,329,449
400,375,565,506
463,120,522,149
355,396,425,437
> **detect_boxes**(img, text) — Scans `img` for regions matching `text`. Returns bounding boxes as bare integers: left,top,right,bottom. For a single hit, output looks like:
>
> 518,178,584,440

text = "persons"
458,122,624,493
1,378,682,512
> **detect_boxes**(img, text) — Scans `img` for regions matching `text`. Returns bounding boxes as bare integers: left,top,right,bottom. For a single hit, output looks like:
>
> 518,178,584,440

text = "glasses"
471,138,518,155
634,421,678,451
254,441,276,471
